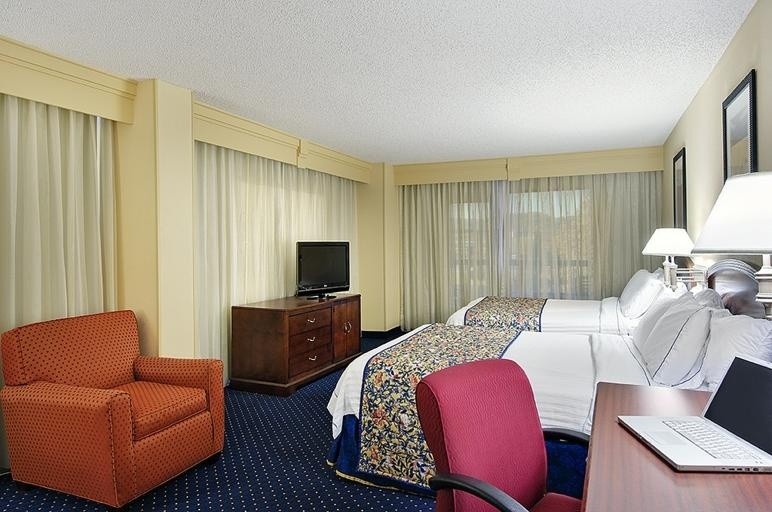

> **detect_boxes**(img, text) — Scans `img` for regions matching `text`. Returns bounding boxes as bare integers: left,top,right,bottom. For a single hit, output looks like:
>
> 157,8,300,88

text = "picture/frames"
673,147,687,232
722,68,758,184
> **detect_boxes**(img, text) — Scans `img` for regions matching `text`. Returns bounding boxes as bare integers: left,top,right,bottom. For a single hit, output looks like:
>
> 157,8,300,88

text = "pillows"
616,269,771,386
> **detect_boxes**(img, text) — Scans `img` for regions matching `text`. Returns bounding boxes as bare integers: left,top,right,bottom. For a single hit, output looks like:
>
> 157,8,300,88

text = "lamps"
642,228,695,287
691,170,771,254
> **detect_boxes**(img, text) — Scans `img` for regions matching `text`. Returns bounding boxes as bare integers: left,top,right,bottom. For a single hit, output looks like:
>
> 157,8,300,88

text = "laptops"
616,353,772,475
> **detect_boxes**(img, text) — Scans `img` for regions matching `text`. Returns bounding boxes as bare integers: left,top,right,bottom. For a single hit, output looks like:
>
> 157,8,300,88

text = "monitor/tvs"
296,241,350,299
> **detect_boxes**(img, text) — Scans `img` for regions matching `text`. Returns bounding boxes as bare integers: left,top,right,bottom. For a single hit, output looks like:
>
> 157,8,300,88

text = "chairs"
414,360,590,511
1,310,225,509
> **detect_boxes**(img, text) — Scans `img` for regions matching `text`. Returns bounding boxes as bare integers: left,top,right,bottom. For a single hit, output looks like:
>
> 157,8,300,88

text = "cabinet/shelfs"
232,294,362,398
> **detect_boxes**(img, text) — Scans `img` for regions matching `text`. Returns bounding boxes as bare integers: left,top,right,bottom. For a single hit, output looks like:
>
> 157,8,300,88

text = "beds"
328,323,651,494
446,295,625,335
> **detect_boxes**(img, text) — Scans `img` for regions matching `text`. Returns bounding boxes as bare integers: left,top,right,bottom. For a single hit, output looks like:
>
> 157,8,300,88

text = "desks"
586,382,771,512
